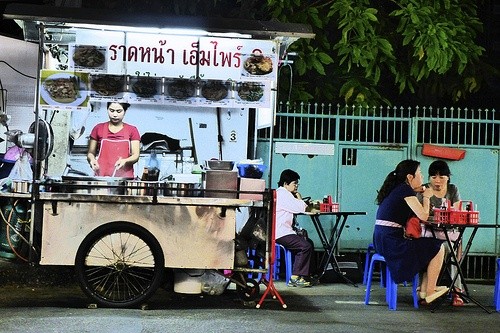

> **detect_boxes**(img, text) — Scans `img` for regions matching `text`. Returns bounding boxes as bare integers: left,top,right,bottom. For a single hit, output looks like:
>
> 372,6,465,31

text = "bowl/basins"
236,162,265,178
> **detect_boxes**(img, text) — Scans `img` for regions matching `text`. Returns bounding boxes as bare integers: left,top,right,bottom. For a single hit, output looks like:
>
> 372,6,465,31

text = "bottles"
465,204,471,213
442,203,446,211
323,196,328,204
141,168,148,180
148,149,160,181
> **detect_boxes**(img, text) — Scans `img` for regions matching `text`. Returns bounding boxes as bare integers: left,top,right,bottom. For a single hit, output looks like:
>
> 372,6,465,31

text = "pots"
12,179,33,194
207,158,235,170
162,181,187,197
125,180,159,197
185,183,205,197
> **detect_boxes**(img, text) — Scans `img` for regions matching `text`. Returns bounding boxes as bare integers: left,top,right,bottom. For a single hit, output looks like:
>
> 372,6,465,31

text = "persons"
372,159,449,303
416,160,465,305
274,168,315,288
87,103,141,179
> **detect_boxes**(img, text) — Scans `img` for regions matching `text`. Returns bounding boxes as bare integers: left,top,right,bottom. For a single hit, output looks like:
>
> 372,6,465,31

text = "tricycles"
0,14,317,308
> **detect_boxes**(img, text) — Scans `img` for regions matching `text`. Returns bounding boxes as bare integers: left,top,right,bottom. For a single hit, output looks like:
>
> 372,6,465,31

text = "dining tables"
420,220,500,314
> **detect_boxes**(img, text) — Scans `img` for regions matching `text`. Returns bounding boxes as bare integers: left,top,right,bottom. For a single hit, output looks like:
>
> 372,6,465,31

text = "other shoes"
453,291,464,307
287,275,313,288
419,286,447,299
424,288,449,303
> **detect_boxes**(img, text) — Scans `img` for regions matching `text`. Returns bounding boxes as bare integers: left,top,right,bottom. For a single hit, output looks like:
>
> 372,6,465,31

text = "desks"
301,210,366,287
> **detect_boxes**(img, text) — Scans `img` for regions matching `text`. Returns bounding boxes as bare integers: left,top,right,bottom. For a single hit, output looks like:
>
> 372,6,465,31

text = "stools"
362,243,407,288
365,253,420,311
247,242,294,284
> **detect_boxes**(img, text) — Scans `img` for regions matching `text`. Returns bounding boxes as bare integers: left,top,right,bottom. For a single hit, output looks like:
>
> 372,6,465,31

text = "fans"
19,119,54,190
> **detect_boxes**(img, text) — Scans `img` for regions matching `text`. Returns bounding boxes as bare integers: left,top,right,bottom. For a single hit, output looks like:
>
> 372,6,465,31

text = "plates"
41,73,87,106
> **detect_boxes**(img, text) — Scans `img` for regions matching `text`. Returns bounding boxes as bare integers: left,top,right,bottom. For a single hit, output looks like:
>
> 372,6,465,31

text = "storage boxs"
433,207,479,225
238,178,265,200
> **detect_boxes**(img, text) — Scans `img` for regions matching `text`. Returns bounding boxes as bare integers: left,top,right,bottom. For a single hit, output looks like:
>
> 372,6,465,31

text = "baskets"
433,210,479,224
320,203,339,212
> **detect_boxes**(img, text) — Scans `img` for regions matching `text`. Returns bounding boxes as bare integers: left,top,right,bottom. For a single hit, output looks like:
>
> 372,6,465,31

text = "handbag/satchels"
405,217,422,238
